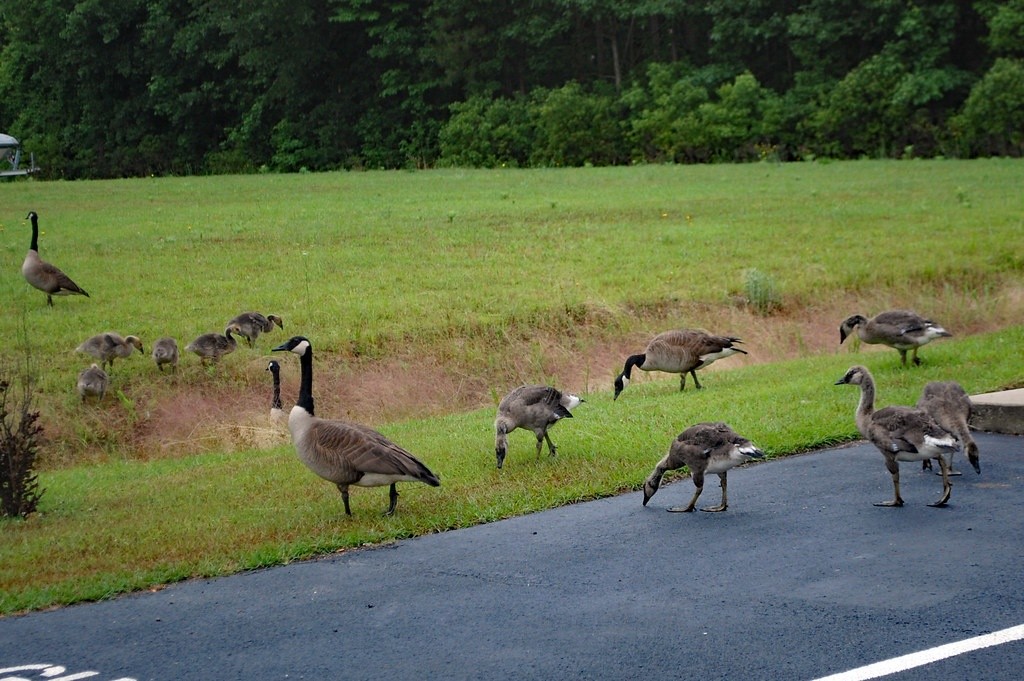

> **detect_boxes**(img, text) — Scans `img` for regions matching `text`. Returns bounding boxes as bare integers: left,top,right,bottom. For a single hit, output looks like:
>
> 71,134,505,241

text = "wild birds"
840,310,953,367
497,385,588,467
23,211,284,407
269,336,439,514
835,365,960,507
266,361,287,425
916,381,981,475
643,423,765,512
612,330,748,400
